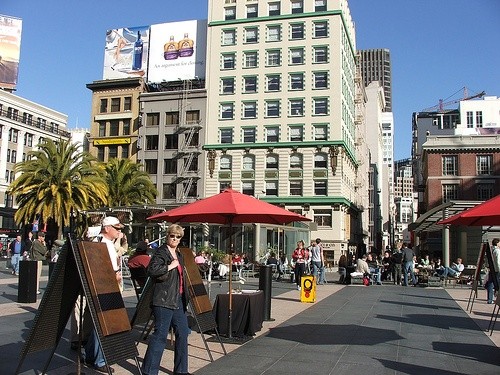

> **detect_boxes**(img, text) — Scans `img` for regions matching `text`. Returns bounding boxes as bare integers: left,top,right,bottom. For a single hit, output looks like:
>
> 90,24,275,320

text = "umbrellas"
148,187,314,342
436,194,500,228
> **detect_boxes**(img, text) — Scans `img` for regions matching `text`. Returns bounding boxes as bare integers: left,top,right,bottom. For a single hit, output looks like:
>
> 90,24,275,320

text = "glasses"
111,225,123,230
169,234,182,239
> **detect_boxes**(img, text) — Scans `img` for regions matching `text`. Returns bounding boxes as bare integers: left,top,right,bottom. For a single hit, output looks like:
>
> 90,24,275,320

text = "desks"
211,289,266,338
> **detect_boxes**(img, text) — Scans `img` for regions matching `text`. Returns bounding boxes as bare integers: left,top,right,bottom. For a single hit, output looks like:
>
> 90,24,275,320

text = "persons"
486,238,500,304
293,238,326,292
7,229,49,295
128,241,153,287
338,242,466,289
144,238,151,249
84,215,124,371
195,247,291,283
142,224,194,375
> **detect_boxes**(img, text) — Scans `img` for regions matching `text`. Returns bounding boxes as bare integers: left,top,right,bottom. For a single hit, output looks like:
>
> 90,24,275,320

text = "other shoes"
80,359,87,365
298,285,301,290
174,372,191,375
488,300,495,304
12,271,14,274
437,274,444,281
15,272,19,275
87,363,114,373
467,280,473,285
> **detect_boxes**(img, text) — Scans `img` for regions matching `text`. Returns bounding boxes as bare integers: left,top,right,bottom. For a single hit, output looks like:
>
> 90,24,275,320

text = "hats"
272,253,276,256
38,232,46,236
102,216,125,228
137,242,148,251
